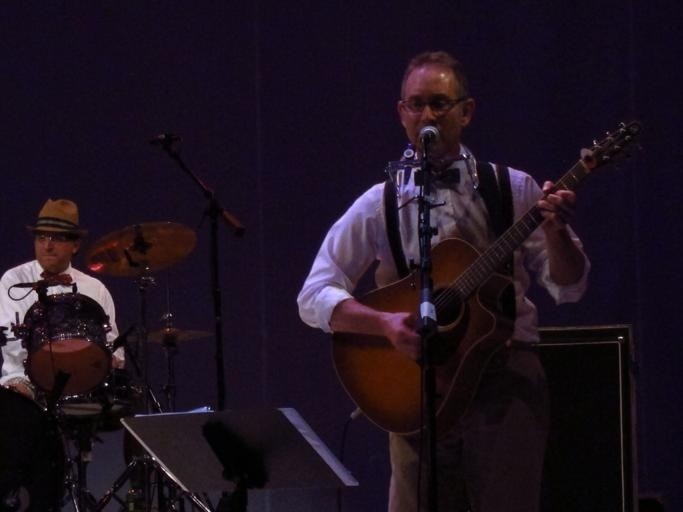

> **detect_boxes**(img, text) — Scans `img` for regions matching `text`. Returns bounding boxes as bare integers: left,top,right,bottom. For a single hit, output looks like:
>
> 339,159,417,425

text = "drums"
20,295,111,398
0,386,79,511
51,367,137,417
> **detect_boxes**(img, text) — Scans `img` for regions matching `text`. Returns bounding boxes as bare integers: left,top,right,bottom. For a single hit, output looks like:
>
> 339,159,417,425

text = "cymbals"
85,221,196,276
119,328,213,346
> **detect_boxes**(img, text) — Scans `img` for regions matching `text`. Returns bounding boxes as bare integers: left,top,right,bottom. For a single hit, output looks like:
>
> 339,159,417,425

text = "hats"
25,198,88,236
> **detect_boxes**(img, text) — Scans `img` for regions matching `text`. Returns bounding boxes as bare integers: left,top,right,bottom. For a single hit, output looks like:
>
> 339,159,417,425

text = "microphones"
418,125,441,144
148,130,169,148
13,277,73,292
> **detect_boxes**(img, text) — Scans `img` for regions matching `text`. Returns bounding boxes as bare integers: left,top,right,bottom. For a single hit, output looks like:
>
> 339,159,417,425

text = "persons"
297,50,592,511
0,198,126,512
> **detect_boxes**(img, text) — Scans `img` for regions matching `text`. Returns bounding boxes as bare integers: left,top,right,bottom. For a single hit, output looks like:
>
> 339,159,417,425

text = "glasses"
401,98,466,112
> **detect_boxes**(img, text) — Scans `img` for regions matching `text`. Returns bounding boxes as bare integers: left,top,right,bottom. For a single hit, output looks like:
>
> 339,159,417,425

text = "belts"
507,340,537,350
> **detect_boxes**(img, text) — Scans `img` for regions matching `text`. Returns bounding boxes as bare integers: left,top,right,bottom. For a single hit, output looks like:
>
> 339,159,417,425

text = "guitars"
329,121,644,441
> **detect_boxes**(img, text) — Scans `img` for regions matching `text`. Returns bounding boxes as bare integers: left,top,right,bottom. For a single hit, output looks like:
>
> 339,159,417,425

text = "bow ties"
40,272,73,286
414,168,460,186
